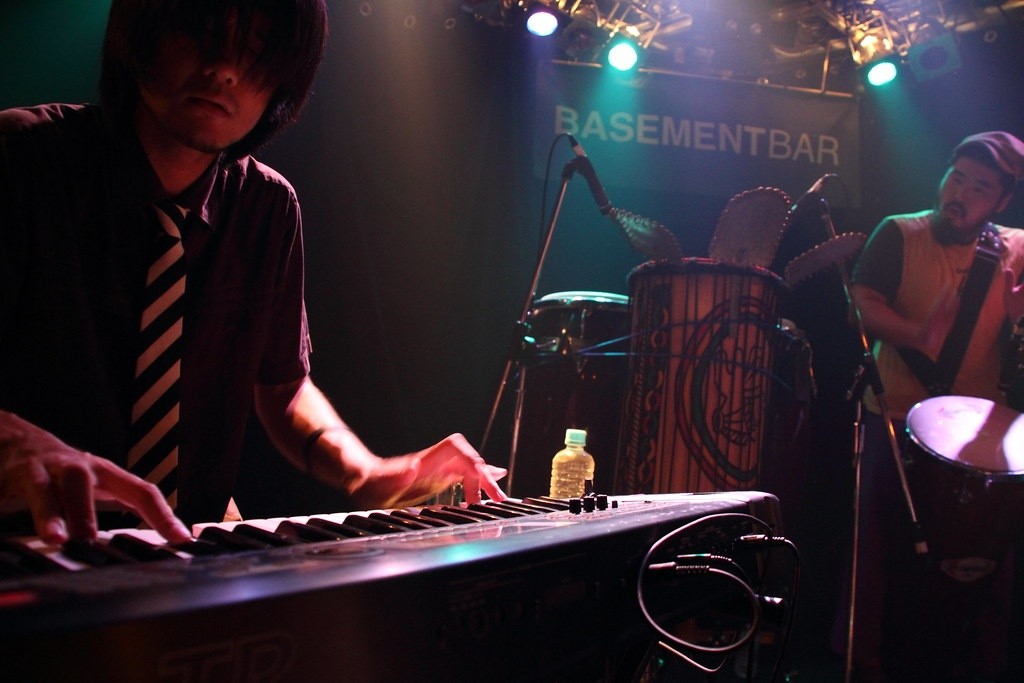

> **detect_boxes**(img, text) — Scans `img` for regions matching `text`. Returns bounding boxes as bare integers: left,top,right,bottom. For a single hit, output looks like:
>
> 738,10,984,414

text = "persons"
1,0,511,550
844,128,1023,681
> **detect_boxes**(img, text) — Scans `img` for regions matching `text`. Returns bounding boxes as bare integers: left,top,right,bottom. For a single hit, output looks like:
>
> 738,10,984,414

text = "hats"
953,130,1023,179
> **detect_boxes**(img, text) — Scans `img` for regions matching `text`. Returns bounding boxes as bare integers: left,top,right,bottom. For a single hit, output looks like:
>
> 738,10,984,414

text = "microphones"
567,132,614,216
787,172,833,235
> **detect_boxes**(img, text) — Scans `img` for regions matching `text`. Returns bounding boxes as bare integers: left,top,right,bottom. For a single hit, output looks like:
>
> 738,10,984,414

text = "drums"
610,254,789,493
529,289,633,385
901,392,1024,545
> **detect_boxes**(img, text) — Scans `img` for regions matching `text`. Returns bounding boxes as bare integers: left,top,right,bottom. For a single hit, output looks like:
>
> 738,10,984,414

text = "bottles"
550,429,595,499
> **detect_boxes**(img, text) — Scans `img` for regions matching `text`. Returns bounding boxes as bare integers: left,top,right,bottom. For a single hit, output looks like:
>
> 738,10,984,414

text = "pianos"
0,489,789,683
